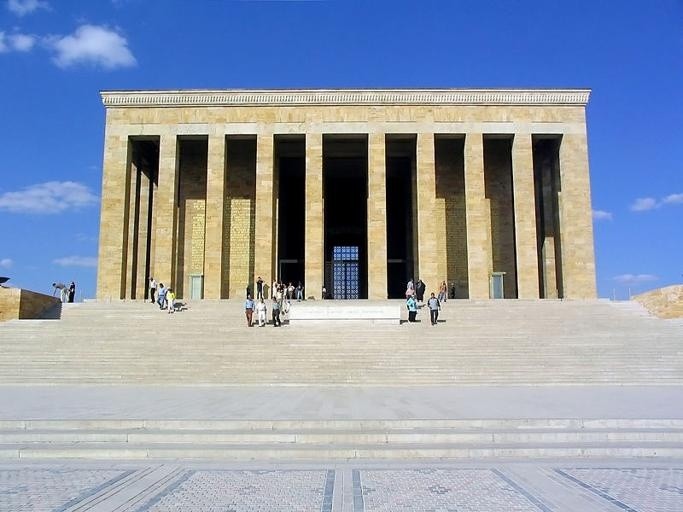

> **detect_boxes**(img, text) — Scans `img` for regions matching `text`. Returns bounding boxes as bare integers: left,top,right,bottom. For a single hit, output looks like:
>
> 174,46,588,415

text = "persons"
67,282,75,302
404,277,446,326
450,283,456,299
244,276,304,327
53,282,68,303
322,286,327,299
149,278,157,303
156,282,176,314
